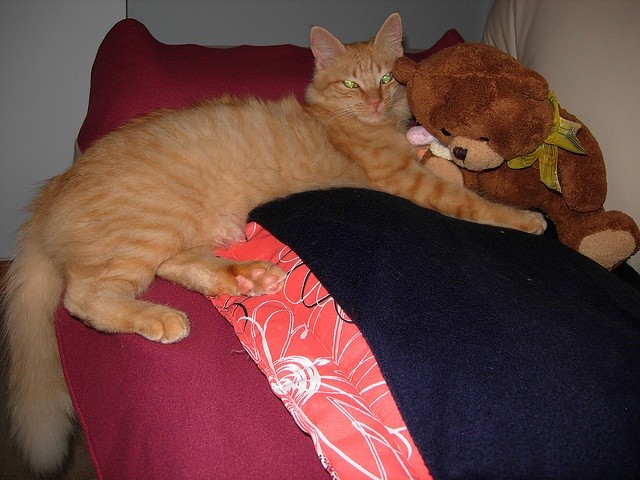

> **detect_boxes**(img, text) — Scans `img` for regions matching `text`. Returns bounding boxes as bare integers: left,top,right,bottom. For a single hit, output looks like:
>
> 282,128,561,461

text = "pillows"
204,220,431,479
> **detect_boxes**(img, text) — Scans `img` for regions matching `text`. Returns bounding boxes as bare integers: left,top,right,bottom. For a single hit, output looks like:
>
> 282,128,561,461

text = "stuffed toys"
392,43,640,272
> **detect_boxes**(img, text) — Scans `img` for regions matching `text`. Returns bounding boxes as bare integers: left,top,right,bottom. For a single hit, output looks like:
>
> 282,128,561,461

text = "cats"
2,12,548,480
